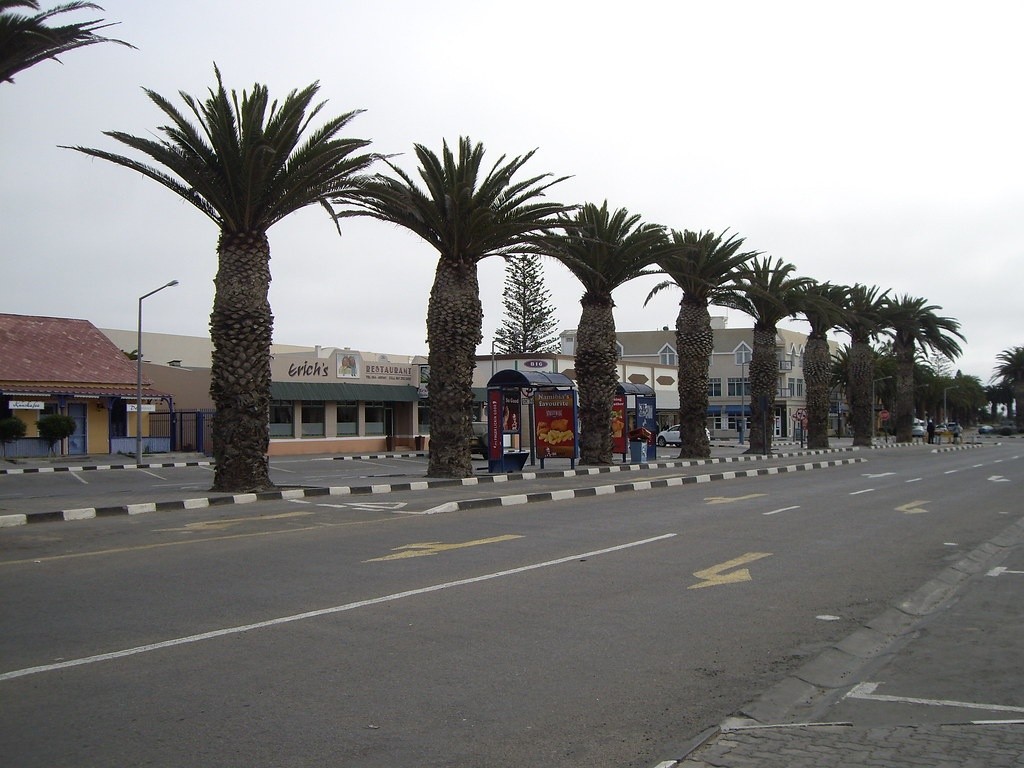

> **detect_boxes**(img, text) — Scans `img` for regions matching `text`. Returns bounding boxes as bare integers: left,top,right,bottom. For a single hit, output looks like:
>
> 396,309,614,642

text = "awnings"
0,384,175,402
268,381,422,403
706,405,752,412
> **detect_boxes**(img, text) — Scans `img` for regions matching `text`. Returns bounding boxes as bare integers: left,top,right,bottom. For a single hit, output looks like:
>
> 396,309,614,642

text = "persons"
927,419,935,444
952,423,961,444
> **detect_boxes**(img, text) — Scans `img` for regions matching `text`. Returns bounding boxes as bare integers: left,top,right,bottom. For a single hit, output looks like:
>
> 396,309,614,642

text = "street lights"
943,385,960,425
135,278,180,462
490,330,525,376
872,376,893,439
739,359,749,443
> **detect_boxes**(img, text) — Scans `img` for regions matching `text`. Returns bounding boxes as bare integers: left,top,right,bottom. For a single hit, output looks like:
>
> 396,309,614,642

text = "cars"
656,423,711,448
978,425,994,434
934,424,949,433
912,424,926,437
